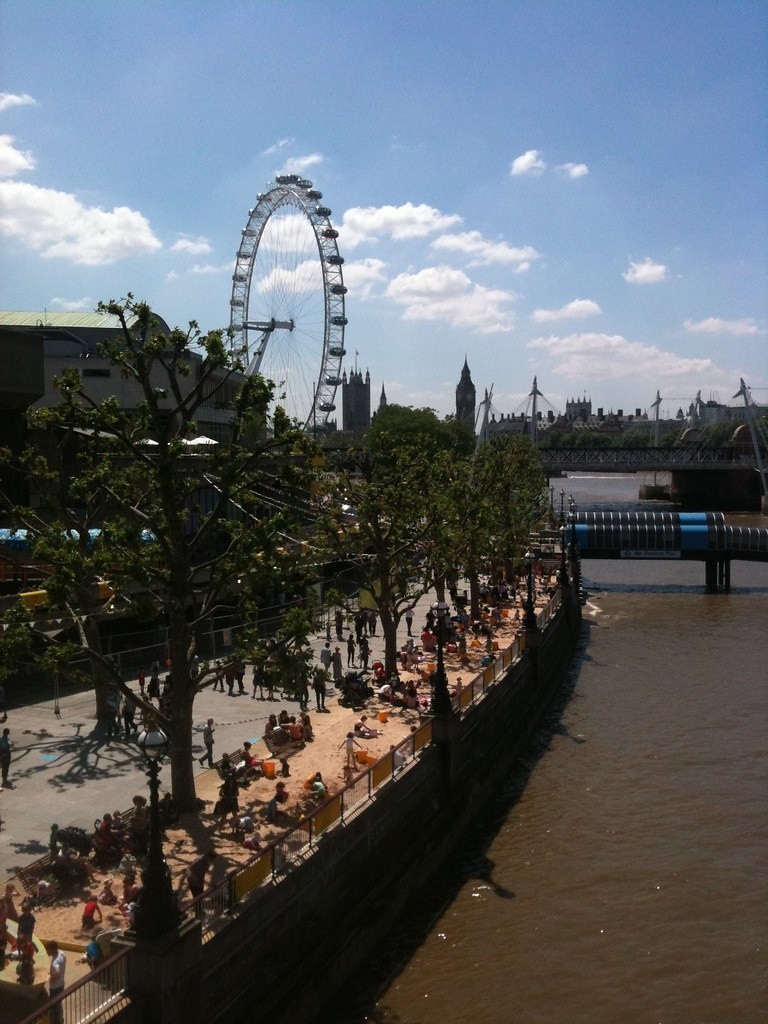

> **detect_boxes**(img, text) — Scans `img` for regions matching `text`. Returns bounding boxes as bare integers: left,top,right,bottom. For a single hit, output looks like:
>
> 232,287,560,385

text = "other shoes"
208,763,213,766
329,629,419,671
140,692,146,695
96,870,102,874
126,728,138,736
216,833,223,837
2,715,7,719
246,778,251,783
93,878,101,883
109,728,119,737
3,777,10,784
199,759,203,765
199,687,311,710
136,852,147,858
318,705,326,709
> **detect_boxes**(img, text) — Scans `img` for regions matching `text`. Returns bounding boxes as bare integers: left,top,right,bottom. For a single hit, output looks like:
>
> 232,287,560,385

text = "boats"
639,486,672,501
548,469,568,479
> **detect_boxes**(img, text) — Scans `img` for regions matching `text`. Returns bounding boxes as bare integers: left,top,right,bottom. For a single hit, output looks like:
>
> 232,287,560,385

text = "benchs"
88,807,143,863
262,717,307,757
15,846,91,910
215,749,261,781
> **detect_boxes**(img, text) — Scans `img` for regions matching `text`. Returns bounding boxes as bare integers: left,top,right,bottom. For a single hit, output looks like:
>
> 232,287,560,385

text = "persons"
1,547,562,1024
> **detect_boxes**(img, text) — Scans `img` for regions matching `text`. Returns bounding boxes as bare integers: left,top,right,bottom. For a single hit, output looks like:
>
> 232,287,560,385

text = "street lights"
559,526,570,583
428,596,454,715
548,485,554,514
523,546,538,627
570,511,576,549
560,488,566,523
133,713,180,928
570,500,578,514
567,494,574,513
